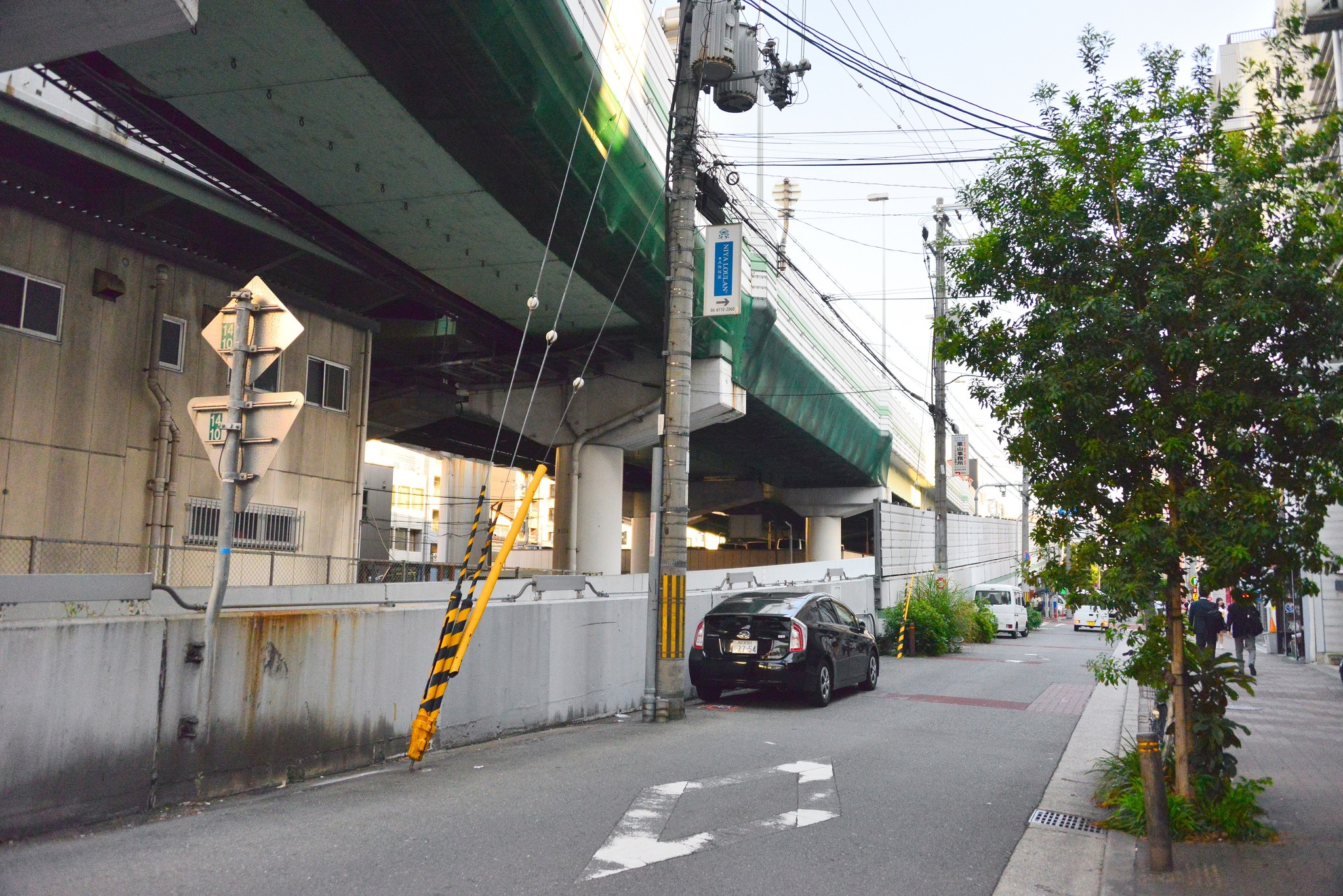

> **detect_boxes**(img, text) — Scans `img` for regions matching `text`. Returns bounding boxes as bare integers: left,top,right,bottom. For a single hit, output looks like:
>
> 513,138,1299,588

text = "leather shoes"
1237,670,1244,674
1248,663,1256,676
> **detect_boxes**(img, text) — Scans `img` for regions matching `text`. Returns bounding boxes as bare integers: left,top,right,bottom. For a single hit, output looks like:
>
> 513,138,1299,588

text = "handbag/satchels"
1038,601,1044,610
1253,621,1264,636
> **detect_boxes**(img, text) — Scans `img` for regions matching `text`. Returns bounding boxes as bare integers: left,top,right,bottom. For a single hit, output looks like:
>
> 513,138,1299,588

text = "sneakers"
1220,644,1224,649
1215,642,1219,648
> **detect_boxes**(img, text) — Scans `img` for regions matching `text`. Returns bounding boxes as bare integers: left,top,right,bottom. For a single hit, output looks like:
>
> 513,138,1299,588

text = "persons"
1214,597,1227,650
1187,586,1224,661
1031,592,1043,612
1225,587,1261,676
1180,596,1195,615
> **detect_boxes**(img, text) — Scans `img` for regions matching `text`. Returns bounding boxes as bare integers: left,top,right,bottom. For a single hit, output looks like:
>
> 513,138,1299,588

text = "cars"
688,591,882,704
1040,604,1068,616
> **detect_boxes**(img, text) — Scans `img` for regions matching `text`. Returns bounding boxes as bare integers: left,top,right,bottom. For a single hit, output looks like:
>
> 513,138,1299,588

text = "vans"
1074,588,1110,632
972,584,1030,638
1047,594,1068,608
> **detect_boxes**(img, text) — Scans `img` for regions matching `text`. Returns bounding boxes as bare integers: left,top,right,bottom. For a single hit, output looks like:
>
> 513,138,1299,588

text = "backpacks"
1205,604,1225,634
1181,601,1189,614
